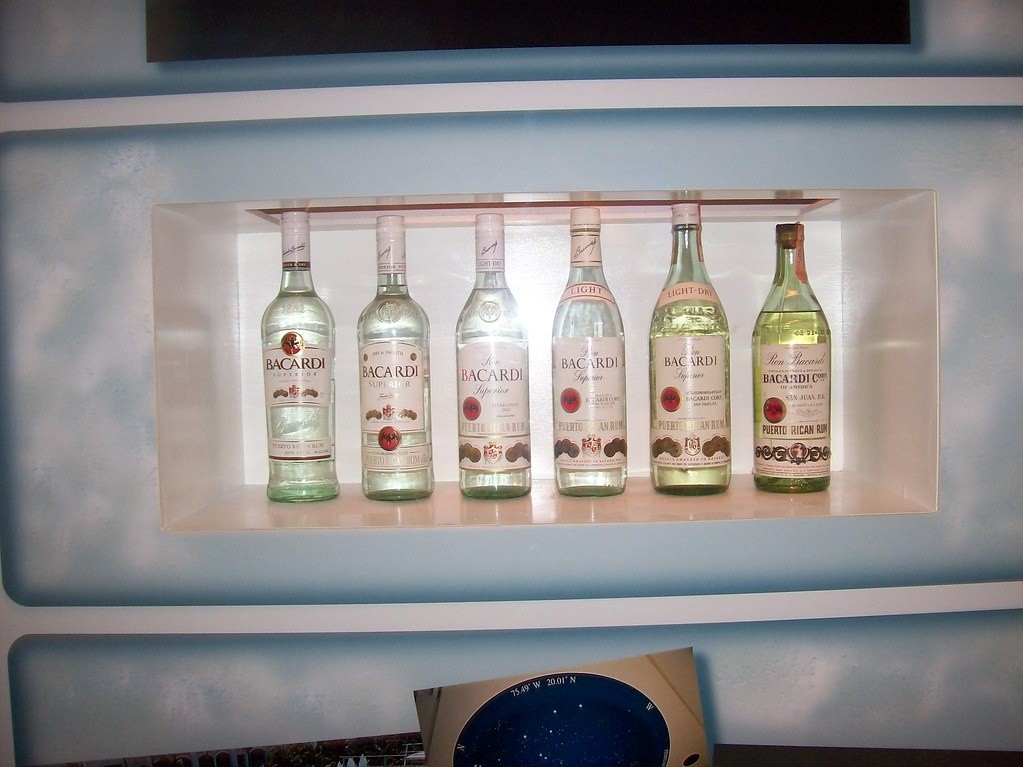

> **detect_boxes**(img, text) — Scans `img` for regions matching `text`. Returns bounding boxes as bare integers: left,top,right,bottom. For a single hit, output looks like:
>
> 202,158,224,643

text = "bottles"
260,210,340,504
750,221,833,494
454,213,532,500
355,214,434,501
550,207,628,498
647,203,733,497
